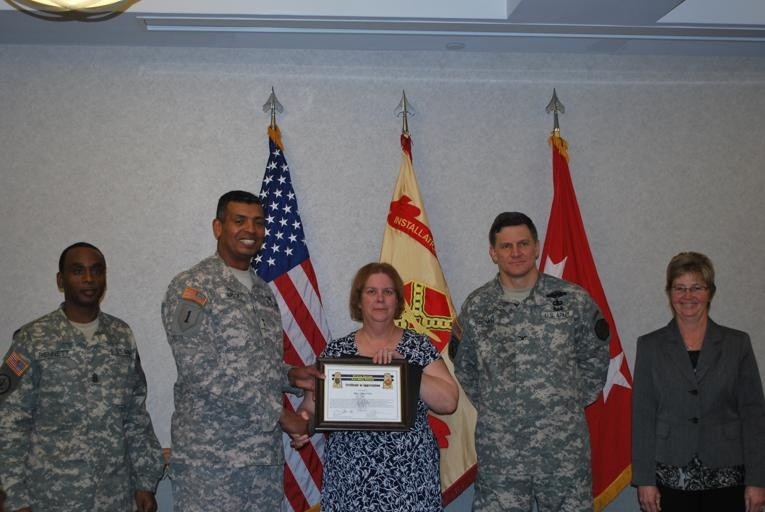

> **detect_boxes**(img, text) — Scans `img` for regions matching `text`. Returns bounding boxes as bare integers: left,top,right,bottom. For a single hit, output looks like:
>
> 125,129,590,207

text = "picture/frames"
314,357,409,432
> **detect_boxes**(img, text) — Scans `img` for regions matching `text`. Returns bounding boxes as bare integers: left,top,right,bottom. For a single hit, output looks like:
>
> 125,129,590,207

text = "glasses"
672,284,709,292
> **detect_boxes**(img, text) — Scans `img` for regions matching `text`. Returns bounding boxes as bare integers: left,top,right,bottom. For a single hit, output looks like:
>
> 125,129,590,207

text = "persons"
288,263,459,512
448,211,611,512
631,251,765,512
0,241,166,512
160,191,324,512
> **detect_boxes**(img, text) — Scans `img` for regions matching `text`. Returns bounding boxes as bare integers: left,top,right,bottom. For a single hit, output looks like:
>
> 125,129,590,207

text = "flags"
379,90,478,507
248,86,332,512
537,88,633,512
